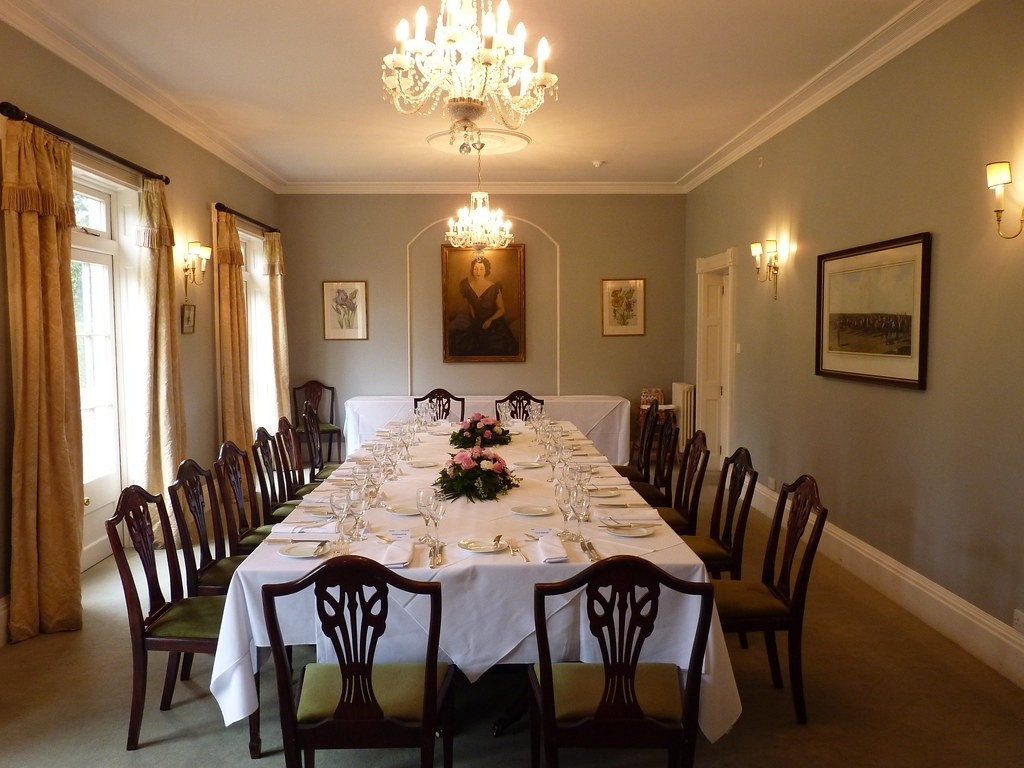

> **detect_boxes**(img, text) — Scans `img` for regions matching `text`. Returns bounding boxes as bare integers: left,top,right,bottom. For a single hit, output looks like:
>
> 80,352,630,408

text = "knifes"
580,542,595,562
597,523,662,528
596,503,648,508
493,535,502,548
312,541,327,554
428,546,435,568
587,542,600,560
436,546,442,564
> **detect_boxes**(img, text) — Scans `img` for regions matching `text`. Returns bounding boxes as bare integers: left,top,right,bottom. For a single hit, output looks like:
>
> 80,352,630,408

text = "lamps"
183,241,211,301
987,161,1024,240
750,239,779,300
381,0,556,250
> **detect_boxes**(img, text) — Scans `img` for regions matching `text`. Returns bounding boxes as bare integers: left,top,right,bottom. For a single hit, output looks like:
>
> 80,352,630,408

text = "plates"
384,505,419,516
589,489,620,497
513,462,544,468
276,542,330,558
510,505,554,515
606,527,655,537
406,460,437,468
458,538,508,553
560,432,599,474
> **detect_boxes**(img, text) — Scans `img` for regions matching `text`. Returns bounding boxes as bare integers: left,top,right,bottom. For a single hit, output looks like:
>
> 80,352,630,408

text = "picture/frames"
601,278,646,336
321,281,368,340
440,242,528,363
182,304,195,333
814,232,931,391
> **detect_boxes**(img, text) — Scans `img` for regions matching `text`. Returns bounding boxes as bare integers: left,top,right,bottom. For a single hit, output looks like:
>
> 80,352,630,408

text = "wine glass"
329,403,447,547
496,402,590,541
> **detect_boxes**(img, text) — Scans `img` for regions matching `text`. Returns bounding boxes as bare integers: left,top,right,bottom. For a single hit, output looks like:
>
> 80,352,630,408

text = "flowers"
447,412,512,449
435,445,522,504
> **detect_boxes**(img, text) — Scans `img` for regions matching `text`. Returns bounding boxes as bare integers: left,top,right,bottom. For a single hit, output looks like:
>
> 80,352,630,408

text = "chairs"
496,390,545,421
611,401,830,725
101,380,361,758
534,552,714,768
413,389,466,422
261,556,452,767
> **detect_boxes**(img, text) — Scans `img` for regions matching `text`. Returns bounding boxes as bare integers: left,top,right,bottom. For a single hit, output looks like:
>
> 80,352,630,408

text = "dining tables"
205,419,745,742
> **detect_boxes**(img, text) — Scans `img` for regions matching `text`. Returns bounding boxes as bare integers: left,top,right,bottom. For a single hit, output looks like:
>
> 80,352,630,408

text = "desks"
344,396,630,467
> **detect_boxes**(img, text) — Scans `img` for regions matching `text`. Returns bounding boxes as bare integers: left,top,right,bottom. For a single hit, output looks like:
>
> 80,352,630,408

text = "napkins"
263,419,663,571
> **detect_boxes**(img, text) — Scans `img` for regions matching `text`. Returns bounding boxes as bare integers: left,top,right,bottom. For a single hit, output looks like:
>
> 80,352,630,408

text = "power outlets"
1013,609,1024,633
